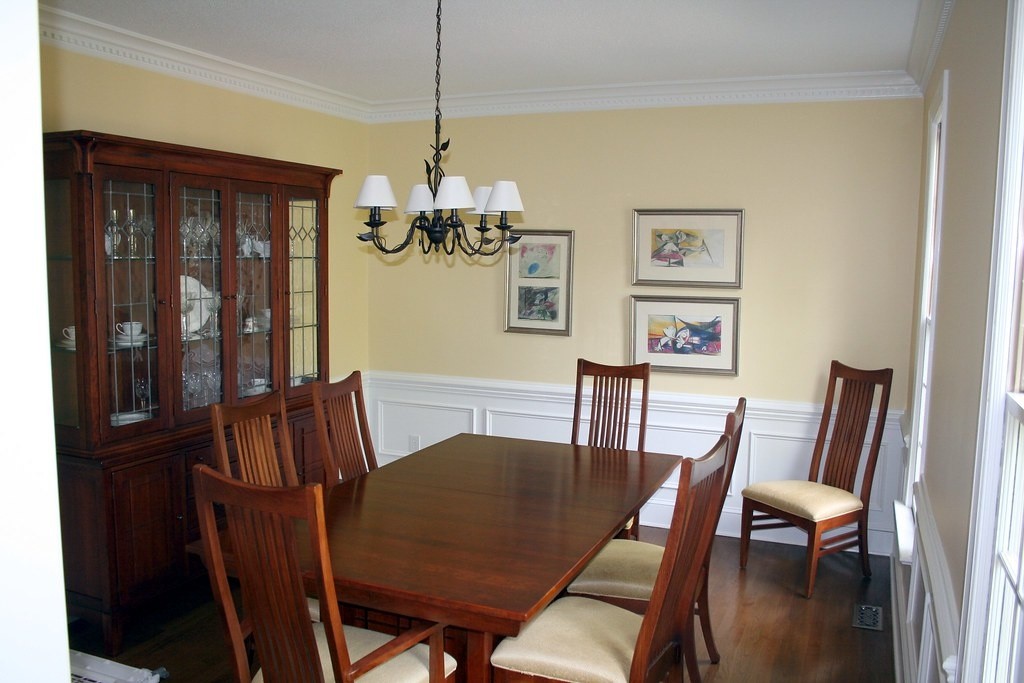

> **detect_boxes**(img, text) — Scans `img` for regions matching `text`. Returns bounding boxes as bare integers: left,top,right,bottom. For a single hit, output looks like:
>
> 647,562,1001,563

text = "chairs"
490,434,731,683
741,359,894,598
191,463,457,683
312,371,379,488
212,391,300,487
570,358,651,540
567,397,746,683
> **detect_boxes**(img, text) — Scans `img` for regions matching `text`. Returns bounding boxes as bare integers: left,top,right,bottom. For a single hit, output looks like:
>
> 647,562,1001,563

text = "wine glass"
183,371,221,410
136,379,149,419
181,291,220,340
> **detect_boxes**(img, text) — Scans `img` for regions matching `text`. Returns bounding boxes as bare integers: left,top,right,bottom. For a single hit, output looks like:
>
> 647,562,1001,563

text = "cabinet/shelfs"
43,131,342,658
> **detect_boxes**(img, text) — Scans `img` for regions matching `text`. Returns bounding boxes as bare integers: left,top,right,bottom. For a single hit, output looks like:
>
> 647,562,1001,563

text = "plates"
181,275,210,333
62,341,75,345
111,413,152,426
117,334,146,339
56,345,75,348
246,388,271,396
110,338,151,342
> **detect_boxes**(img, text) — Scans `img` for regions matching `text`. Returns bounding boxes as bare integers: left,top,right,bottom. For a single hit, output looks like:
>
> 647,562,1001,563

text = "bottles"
110,210,118,256
127,209,137,256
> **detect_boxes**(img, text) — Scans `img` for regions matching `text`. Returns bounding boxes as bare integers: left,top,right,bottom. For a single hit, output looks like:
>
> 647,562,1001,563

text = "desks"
184,433,683,683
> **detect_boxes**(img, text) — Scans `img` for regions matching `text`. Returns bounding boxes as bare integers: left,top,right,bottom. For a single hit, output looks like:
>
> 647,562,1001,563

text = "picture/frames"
628,294,740,377
505,229,575,336
632,208,744,290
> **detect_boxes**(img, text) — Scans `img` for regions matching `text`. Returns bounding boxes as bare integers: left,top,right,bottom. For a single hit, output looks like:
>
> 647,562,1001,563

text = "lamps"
354,0,525,256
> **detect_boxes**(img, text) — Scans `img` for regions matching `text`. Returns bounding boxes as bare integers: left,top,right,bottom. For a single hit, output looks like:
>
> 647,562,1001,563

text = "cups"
252,379,267,390
116,322,143,336
63,326,75,340
